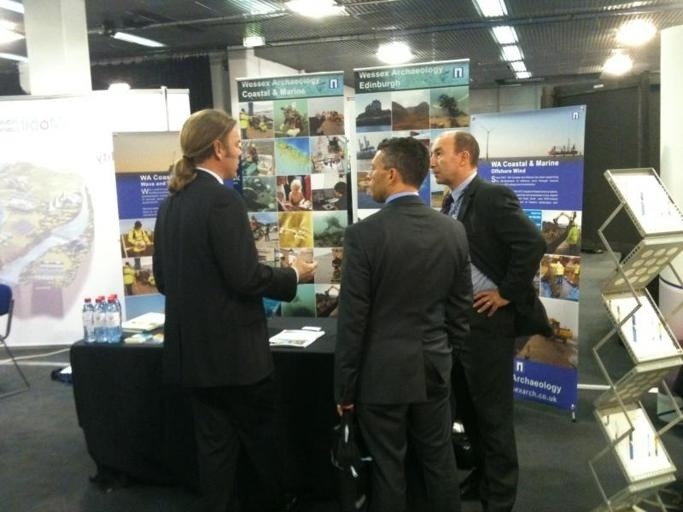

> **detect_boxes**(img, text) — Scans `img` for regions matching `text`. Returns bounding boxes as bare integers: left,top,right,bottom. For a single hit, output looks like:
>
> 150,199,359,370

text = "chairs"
0,284,29,398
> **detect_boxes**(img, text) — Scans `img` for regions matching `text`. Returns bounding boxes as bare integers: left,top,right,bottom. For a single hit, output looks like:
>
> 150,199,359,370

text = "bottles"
82,293,123,345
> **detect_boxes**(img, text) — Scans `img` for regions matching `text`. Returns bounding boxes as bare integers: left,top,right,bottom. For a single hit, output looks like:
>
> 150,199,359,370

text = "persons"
429,130,550,512
237,143,261,176
334,181,347,210
545,222,581,288
127,220,150,256
238,108,256,139
334,137,476,512
148,108,318,511
287,180,304,206
123,262,135,295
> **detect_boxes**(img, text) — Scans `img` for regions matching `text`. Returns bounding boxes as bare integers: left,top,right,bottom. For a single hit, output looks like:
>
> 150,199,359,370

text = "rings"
487,301,490,305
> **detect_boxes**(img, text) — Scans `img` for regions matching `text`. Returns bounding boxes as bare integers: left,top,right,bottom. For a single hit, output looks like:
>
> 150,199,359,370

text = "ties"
439,191,454,215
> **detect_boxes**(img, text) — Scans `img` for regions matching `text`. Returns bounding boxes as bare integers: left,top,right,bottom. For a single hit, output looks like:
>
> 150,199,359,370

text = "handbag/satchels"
331,411,355,469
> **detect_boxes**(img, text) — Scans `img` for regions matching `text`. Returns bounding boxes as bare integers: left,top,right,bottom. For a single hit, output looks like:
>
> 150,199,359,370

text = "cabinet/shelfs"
584,165,683,512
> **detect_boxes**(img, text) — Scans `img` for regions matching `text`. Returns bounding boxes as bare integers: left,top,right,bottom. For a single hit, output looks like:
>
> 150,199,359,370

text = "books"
120,311,164,333
267,328,325,350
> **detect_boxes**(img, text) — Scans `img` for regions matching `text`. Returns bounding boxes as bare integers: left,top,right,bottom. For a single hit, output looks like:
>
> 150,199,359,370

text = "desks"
69,314,365,511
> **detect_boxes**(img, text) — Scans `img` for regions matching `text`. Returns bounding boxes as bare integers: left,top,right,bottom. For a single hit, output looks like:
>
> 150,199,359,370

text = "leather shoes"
460,481,478,501
